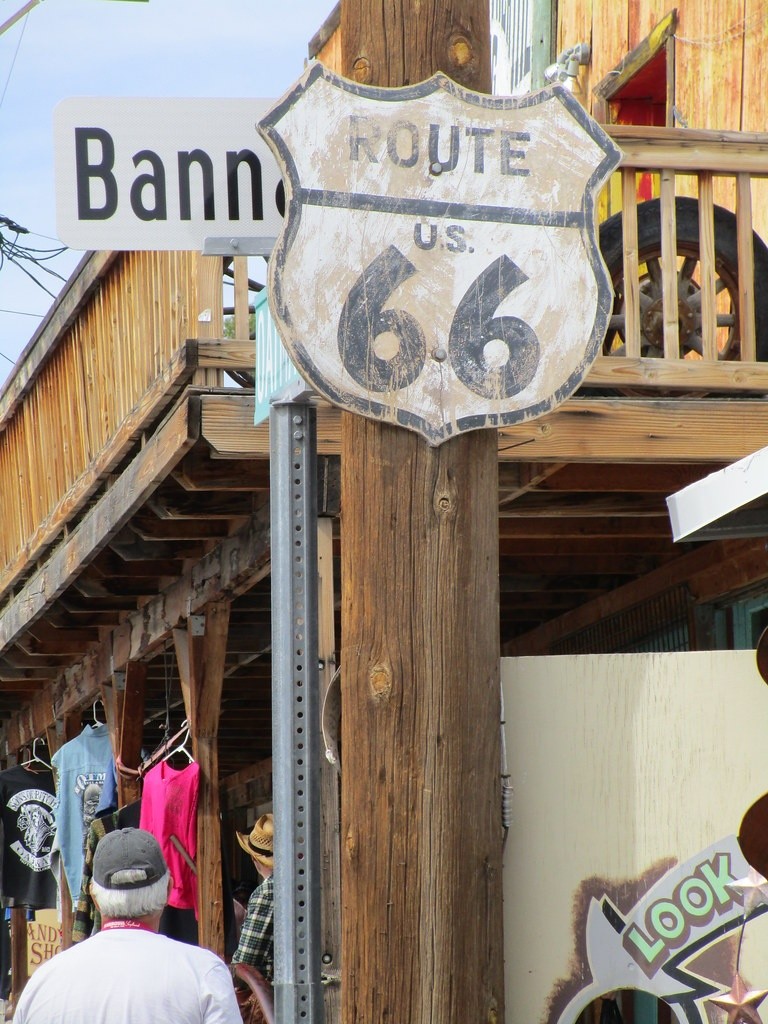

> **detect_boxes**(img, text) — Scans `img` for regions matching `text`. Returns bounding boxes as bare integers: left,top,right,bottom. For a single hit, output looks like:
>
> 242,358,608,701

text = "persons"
231,813,274,1001
10,827,244,1024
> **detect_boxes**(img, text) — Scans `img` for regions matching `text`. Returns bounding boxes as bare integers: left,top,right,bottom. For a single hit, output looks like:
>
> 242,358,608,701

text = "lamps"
543,44,592,96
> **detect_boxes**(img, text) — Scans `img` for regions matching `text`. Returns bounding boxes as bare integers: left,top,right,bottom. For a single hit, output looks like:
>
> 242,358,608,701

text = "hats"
236,813,273,868
92,828,166,890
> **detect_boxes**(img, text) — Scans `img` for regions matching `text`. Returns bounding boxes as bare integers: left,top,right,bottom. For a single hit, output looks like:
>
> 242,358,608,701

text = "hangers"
161,719,195,764
20,738,52,773
89,700,111,729
